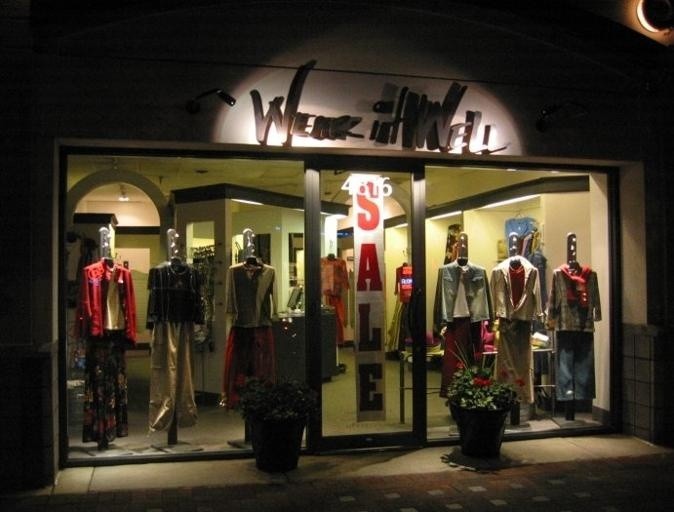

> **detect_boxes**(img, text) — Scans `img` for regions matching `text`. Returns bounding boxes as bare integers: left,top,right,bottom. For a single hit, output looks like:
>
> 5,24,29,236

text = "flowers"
443,339,524,410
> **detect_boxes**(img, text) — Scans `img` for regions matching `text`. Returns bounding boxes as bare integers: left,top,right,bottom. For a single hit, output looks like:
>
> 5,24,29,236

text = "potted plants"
231,375,319,476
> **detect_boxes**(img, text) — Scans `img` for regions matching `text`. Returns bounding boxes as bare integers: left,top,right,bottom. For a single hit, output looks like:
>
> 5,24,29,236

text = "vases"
449,407,511,460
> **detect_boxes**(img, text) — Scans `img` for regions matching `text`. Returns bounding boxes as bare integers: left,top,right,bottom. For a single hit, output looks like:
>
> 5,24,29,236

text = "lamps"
636,1,674,34
